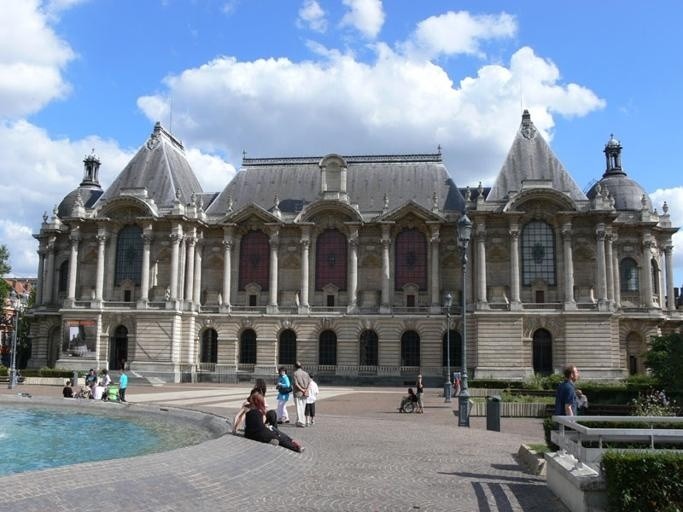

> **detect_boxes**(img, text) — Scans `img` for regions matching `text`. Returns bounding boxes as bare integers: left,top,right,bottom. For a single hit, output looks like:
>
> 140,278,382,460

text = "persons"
62,367,129,404
414,374,424,414
574,388,589,411
452,374,460,398
554,363,586,449
231,359,318,453
399,388,416,414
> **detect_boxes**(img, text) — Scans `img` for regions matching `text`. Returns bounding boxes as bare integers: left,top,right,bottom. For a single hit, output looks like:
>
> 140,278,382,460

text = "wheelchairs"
401,393,420,415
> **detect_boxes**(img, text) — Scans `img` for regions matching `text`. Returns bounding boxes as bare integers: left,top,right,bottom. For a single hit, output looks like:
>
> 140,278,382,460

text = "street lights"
442,289,452,408
454,210,475,430
8,299,21,391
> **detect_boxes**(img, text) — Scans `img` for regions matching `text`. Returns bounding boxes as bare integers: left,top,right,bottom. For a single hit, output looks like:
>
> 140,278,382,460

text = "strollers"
75,386,92,399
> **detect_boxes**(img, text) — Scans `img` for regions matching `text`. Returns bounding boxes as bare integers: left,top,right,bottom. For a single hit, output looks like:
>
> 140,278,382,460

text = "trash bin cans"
484,394,502,432
